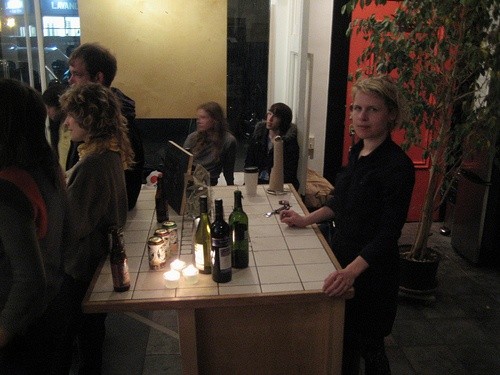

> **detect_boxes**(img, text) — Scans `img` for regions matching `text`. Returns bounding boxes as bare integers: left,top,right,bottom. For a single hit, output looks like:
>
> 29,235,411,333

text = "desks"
82,172,355,375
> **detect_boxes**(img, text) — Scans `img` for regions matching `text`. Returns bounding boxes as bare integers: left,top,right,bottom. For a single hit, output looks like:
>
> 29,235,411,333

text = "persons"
245,102,300,192
183,101,237,186
0,46,145,375
280,74,416,375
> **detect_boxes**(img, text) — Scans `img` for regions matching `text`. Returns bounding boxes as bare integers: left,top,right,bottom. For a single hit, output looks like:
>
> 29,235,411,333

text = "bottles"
212,199,232,283
194,195,212,274
109,229,131,292
229,190,249,269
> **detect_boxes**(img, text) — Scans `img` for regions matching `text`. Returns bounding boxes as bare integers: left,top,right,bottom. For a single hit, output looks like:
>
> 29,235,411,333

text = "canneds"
154,228,169,260
147,236,166,272
161,221,178,253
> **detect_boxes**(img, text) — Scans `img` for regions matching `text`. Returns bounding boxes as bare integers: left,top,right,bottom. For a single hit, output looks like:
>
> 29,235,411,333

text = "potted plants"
341,0,500,301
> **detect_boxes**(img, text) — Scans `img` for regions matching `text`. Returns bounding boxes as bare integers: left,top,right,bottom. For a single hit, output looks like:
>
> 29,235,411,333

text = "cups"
244,167,259,196
